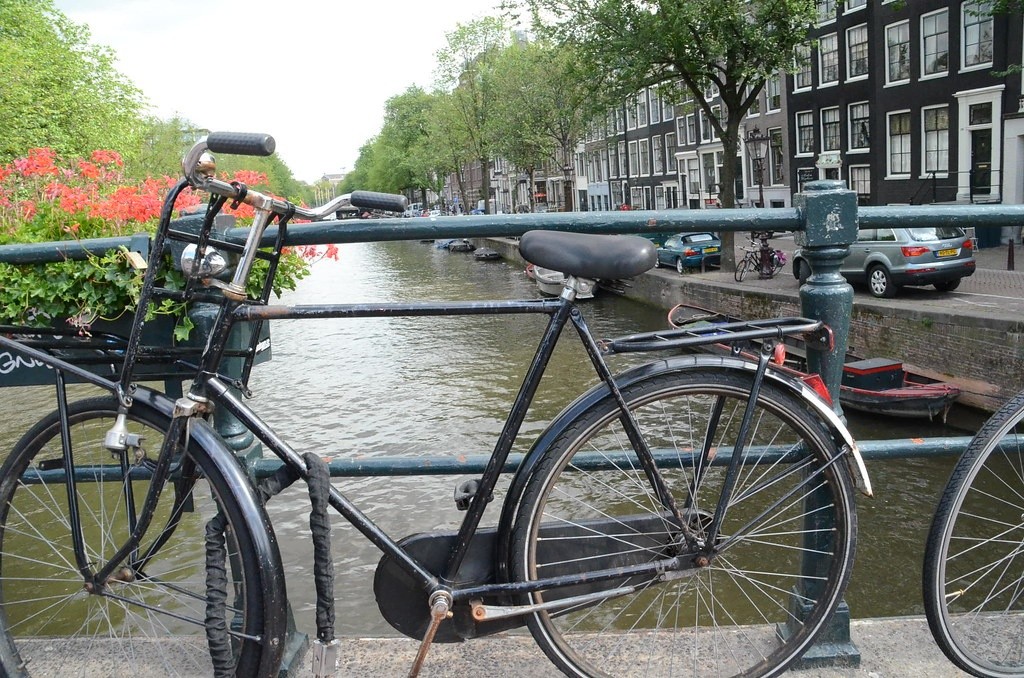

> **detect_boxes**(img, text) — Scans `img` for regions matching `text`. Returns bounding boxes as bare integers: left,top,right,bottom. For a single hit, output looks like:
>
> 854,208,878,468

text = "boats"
473,246,500,261
666,303,963,426
447,238,474,253
526,263,600,301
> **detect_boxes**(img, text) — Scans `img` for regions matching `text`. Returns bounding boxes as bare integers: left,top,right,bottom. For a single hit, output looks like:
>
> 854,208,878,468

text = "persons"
459,203,463,212
442,200,452,211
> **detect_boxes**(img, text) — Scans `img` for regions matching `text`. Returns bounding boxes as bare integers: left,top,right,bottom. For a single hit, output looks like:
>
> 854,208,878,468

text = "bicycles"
920,388,1024,678
1,130,878,678
734,236,783,283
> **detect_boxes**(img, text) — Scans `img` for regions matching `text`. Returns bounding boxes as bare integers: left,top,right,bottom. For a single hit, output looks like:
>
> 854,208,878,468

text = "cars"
792,227,976,299
655,232,721,275
365,198,486,220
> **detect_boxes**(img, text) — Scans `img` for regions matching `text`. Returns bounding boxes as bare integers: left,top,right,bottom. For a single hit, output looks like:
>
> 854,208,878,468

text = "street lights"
744,122,777,281
559,163,575,212
